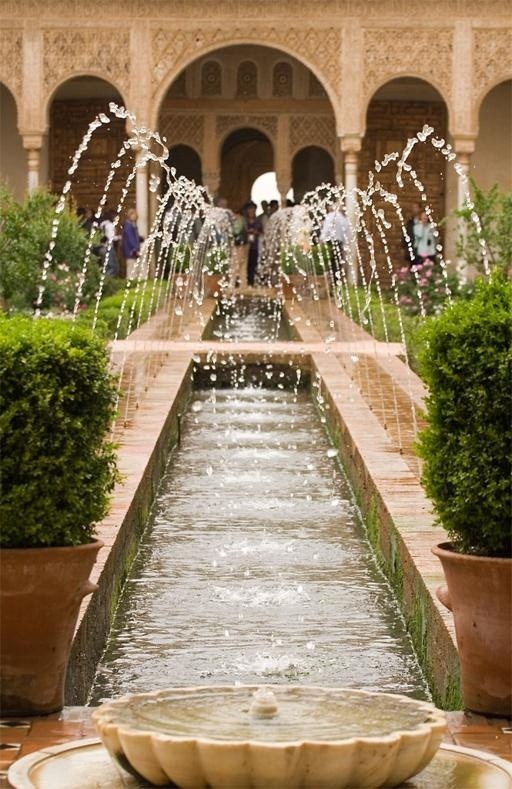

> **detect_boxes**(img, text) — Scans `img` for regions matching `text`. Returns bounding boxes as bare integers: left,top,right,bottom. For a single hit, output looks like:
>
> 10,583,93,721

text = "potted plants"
165,241,230,298
404,264,512,722
277,241,335,301
0,310,131,716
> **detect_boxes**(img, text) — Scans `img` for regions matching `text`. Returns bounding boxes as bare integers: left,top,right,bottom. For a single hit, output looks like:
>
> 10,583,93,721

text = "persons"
413,210,440,274
72,188,347,298
400,201,423,284
